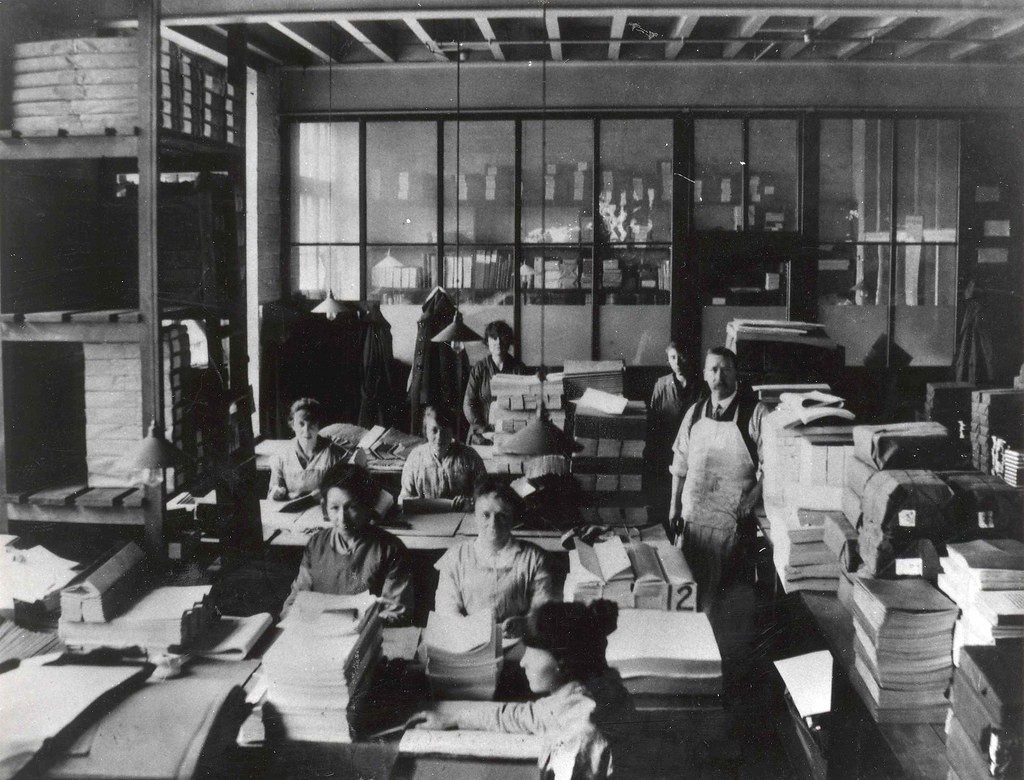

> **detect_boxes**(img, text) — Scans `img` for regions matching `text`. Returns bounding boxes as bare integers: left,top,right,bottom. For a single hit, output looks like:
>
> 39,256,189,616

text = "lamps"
429,18,483,352
311,20,348,321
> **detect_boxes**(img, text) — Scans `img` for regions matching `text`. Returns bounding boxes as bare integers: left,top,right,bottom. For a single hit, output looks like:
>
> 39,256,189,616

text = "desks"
192,444,947,780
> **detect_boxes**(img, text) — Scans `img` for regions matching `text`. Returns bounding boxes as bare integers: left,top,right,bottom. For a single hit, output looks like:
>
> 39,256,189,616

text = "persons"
294,465,414,627
264,399,355,499
433,478,556,702
511,600,656,779
669,344,767,618
397,400,488,513
464,322,528,445
511,460,588,533
650,338,707,429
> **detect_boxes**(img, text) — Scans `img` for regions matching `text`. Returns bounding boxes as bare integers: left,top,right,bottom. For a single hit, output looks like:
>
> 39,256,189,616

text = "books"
59,585,271,661
425,608,503,701
264,489,393,534
357,425,426,461
489,358,723,695
260,591,391,740
727,314,1024,780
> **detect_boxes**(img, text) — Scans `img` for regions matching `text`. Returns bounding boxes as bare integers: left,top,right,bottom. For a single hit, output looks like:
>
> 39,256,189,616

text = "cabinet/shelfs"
0,0,244,569
366,198,759,303
